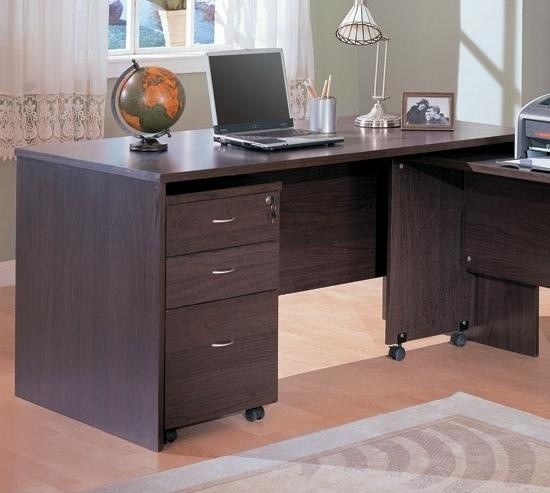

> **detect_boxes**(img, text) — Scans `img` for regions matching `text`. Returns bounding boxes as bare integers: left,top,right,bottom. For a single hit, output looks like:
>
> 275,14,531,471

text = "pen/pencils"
304,73,332,98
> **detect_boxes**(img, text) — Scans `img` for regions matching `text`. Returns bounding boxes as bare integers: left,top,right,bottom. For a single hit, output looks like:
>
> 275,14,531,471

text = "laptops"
203,48,345,152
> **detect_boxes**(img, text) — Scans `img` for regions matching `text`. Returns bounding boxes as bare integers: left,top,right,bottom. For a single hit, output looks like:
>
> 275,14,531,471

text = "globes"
112,58,186,152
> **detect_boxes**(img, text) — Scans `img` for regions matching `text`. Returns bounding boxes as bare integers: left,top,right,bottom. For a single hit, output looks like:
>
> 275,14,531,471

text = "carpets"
81,389,549,493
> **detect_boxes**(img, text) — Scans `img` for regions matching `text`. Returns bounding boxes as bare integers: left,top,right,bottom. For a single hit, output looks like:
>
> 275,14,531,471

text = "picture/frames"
402,91,456,130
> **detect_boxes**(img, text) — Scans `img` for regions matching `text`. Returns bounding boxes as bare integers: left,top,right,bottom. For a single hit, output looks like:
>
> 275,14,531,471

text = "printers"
495,93,550,172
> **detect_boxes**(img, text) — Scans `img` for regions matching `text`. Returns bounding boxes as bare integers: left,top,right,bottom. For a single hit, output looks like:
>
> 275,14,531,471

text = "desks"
463,158,550,356
16,125,515,453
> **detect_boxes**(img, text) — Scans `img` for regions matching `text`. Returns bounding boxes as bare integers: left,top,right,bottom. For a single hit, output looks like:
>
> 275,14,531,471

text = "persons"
407,99,449,125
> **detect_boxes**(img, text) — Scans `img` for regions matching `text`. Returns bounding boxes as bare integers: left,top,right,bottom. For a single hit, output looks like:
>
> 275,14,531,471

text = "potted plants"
148,0,187,48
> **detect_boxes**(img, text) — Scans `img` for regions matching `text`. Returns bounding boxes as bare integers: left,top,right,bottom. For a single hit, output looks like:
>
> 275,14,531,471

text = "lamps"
336,0,402,128
